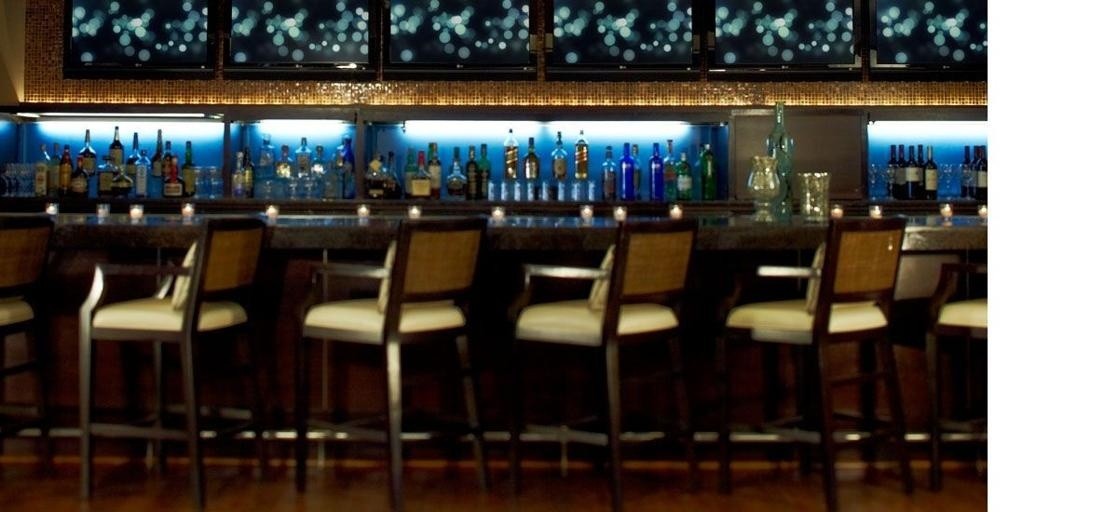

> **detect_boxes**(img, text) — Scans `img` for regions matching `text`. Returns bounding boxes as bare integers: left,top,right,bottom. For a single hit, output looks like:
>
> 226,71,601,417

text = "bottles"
963,143,987,202
765,101,794,214
502,127,590,179
364,140,491,201
602,138,715,200
230,133,356,202
888,142,937,200
34,121,196,199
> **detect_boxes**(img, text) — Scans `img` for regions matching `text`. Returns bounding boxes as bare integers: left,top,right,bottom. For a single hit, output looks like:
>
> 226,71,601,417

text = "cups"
831,203,987,218
798,171,833,222
39,203,687,221
486,179,596,201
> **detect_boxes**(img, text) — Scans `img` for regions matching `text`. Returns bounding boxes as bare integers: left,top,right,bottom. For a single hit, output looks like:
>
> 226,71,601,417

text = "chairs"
0,212,61,481
502,217,705,510
715,217,916,510
75,217,274,511
293,217,494,511
924,259,988,494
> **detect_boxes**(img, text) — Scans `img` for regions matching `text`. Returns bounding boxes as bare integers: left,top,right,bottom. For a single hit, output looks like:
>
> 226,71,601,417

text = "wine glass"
937,160,973,202
2,162,35,198
869,161,899,201
190,165,224,200
